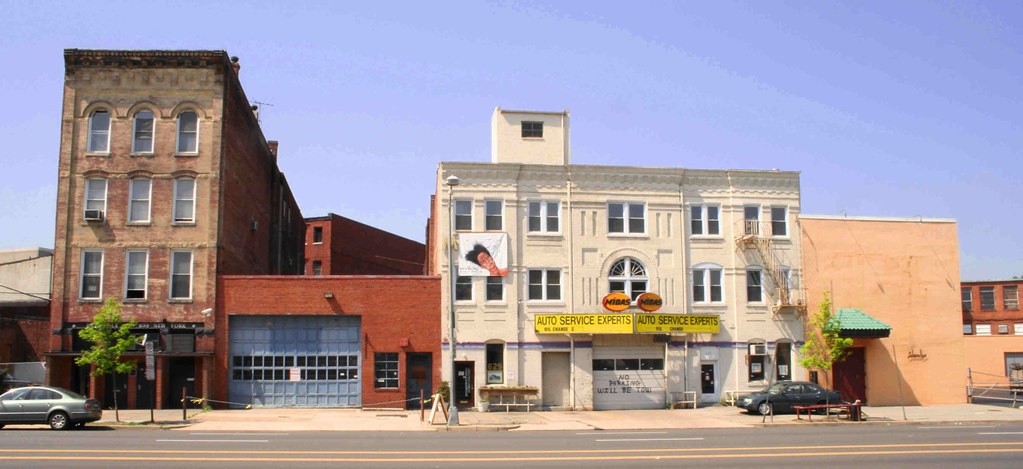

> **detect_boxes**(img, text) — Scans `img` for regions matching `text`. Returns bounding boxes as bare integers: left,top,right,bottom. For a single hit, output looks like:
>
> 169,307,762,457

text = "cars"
0,384,103,431
735,379,843,416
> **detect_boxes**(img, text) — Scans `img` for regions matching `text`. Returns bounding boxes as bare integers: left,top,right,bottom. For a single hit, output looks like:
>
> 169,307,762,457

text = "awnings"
825,308,891,338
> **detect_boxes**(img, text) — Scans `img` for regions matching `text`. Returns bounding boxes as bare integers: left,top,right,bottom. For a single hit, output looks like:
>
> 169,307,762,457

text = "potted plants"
437,381,449,412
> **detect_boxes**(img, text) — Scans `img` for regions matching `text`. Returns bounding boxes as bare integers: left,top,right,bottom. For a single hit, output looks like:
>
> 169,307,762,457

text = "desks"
669,390,697,409
724,390,761,406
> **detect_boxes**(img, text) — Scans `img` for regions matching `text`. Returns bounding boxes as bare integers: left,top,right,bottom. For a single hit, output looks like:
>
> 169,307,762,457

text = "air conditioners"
84,209,104,221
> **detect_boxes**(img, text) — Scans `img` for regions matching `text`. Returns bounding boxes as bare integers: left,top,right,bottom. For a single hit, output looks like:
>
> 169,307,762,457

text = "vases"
677,400,687,409
478,402,489,413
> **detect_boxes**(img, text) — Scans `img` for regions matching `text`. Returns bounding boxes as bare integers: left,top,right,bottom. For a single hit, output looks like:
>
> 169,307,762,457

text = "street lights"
447,175,460,425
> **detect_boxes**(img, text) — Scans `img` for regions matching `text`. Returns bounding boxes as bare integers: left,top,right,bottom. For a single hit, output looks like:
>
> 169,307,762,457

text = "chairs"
789,388,796,394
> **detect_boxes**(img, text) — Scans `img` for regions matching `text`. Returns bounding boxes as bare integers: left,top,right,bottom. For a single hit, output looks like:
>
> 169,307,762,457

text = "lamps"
323,293,334,299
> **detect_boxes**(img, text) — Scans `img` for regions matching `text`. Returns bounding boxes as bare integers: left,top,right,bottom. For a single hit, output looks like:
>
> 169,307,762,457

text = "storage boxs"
749,345,767,355
